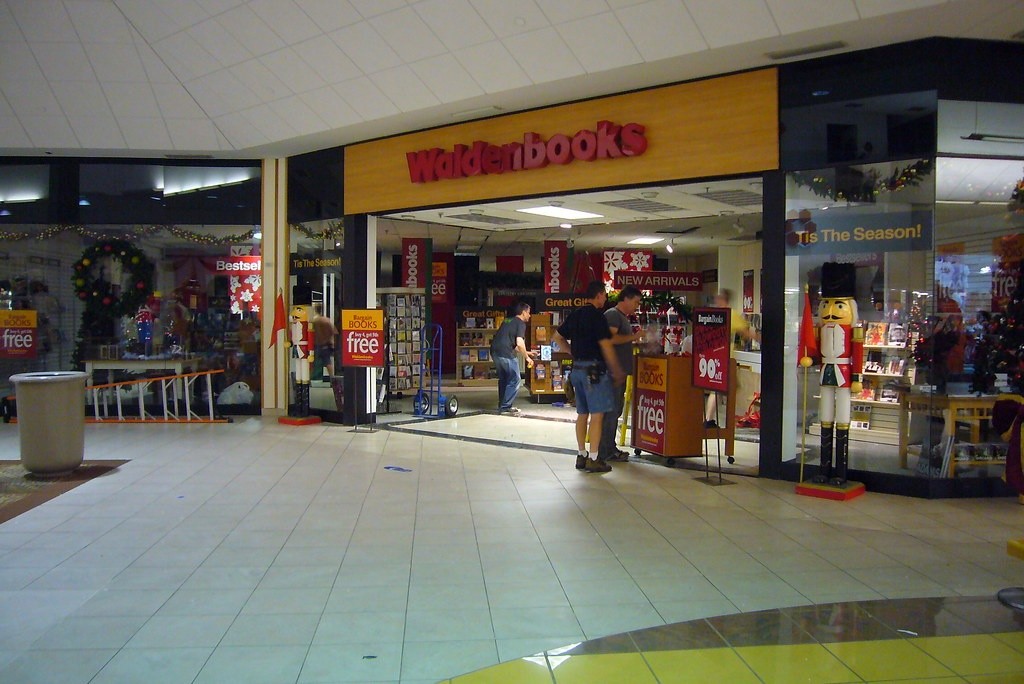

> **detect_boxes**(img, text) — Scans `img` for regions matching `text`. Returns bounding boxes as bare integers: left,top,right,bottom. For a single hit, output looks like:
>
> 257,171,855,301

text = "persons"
706,288,761,429
553,282,625,471
598,285,647,461
800,262,864,484
490,303,533,412
283,285,315,417
312,303,339,387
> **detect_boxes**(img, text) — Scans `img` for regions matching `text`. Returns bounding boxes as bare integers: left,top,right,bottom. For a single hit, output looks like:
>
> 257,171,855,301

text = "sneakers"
576,451,589,468
585,456,612,471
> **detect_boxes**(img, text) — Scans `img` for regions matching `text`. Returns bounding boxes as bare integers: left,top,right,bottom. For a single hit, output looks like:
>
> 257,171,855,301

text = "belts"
575,358,602,361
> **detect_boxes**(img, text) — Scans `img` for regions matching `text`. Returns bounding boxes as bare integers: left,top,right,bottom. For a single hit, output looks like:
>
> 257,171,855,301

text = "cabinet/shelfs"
456,322,496,363
812,345,905,405
531,315,573,393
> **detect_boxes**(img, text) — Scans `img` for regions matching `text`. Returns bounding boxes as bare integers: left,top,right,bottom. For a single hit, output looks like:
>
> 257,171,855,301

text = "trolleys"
413,323,459,420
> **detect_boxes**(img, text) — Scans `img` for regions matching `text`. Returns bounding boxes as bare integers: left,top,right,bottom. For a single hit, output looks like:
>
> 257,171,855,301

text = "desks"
81,358,200,403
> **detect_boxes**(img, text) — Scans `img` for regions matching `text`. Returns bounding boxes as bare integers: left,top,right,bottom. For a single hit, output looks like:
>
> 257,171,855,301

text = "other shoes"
704,420,722,428
608,448,629,461
501,408,521,414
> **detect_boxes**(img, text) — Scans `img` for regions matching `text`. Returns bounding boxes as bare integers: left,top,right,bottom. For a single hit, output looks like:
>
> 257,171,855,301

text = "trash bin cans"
7,370,94,476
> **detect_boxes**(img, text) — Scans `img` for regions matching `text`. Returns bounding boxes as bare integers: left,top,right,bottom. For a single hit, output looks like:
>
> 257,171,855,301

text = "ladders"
2,369,234,424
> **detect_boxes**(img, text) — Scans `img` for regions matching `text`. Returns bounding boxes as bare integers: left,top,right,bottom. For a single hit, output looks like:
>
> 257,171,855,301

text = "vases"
11,371,90,479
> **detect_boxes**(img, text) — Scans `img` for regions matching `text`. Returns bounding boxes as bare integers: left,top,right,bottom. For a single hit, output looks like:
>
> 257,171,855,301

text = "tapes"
527,362,533,369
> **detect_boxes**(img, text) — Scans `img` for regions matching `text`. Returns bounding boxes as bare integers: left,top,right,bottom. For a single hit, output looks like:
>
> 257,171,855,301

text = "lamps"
666,239,678,253
960,99,1024,144
733,217,747,234
